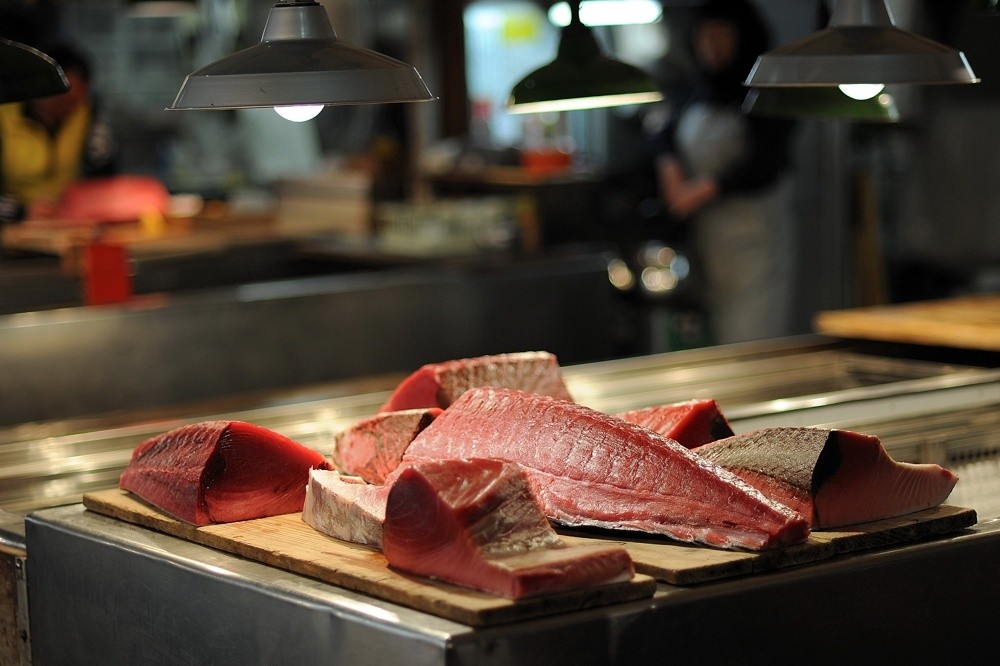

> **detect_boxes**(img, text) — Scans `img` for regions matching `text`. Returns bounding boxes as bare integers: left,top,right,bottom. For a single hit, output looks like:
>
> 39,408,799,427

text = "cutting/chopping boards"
81,484,977,631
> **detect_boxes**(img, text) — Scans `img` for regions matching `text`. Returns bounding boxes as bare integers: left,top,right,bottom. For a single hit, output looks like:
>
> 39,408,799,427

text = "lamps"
506,0,664,113
744,0,980,99
164,0,438,122
